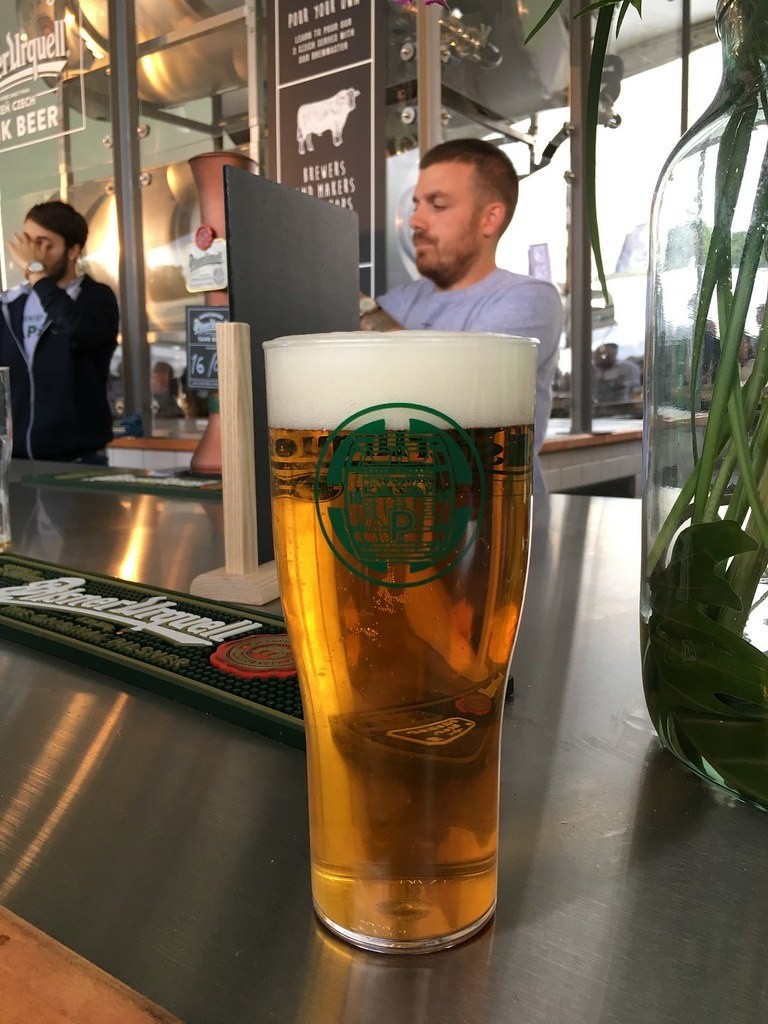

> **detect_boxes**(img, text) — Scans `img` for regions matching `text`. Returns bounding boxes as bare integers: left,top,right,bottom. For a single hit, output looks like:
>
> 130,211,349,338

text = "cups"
0,367,12,547
262,334,543,955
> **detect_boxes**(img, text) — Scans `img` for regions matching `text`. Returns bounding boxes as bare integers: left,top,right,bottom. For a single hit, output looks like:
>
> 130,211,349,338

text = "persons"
0,197,121,466
358,139,565,497
112,359,219,421
591,341,642,417
5,457,131,580
701,299,765,411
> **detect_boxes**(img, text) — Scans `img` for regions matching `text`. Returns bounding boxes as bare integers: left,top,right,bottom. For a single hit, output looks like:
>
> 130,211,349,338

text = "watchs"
25,259,51,279
358,297,383,317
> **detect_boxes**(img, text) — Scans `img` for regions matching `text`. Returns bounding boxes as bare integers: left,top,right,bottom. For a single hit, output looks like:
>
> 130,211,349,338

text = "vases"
638,0,768,810
